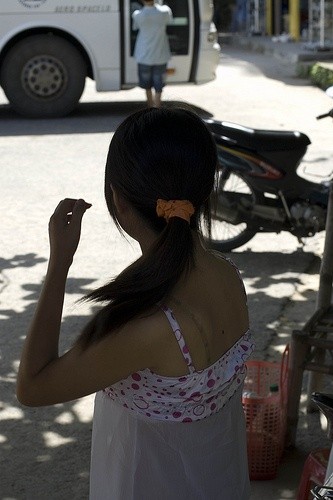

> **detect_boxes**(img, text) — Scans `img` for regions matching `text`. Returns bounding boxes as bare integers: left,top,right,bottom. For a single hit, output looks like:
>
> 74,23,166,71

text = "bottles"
266,384,280,452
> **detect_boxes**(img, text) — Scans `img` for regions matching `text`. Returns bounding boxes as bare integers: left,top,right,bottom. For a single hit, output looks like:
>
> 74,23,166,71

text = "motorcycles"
198,110,332,253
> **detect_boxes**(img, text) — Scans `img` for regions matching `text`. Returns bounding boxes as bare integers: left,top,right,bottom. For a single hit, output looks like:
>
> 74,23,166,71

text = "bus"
1,0,221,116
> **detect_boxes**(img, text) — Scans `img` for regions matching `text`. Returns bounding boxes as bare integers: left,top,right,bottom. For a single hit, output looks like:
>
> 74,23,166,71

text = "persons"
131,0,173,107
17,107,256,500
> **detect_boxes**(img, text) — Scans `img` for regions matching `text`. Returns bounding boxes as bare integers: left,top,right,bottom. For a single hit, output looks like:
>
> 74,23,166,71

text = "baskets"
241,344,289,480
